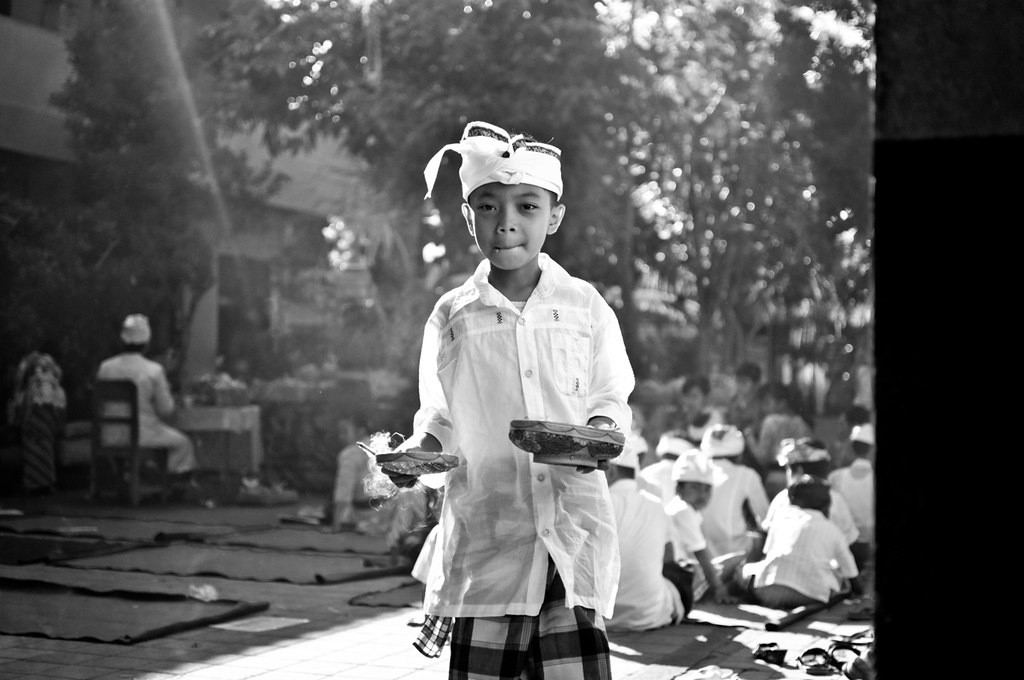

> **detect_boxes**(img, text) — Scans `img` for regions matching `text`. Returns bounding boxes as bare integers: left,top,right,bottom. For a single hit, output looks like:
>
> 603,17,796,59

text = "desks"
162,406,263,500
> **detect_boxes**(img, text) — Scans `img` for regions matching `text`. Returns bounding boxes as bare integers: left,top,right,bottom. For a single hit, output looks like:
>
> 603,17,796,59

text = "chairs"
88,378,169,509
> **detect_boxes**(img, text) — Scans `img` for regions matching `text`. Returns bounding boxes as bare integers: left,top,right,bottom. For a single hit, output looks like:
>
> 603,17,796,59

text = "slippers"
826,640,861,670
797,647,835,675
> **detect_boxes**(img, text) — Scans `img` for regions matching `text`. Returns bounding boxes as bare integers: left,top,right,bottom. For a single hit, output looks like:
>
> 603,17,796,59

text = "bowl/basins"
508,419,626,469
376,451,460,477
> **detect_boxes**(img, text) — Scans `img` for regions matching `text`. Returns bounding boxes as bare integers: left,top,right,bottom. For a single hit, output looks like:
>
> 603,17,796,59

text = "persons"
382,121,635,680
598,363,876,634
13,314,442,567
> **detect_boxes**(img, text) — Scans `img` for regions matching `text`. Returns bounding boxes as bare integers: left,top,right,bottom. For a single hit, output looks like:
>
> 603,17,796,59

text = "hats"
119,313,151,345
672,449,713,485
